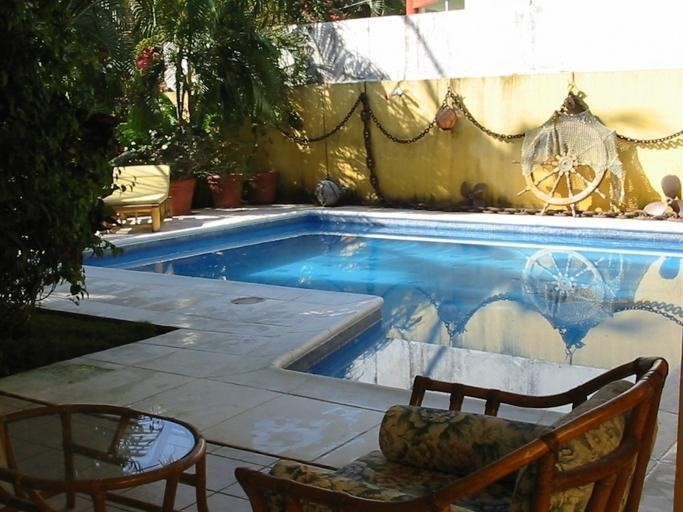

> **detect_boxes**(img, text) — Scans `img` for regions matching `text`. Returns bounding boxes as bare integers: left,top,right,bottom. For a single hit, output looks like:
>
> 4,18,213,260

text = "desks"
0,405,209,512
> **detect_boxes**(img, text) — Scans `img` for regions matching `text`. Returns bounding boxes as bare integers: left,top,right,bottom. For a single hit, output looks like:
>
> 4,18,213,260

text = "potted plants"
106,0,318,216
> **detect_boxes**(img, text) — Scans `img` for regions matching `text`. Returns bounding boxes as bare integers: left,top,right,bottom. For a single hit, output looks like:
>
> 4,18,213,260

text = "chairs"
102,165,173,231
233,355,670,512
